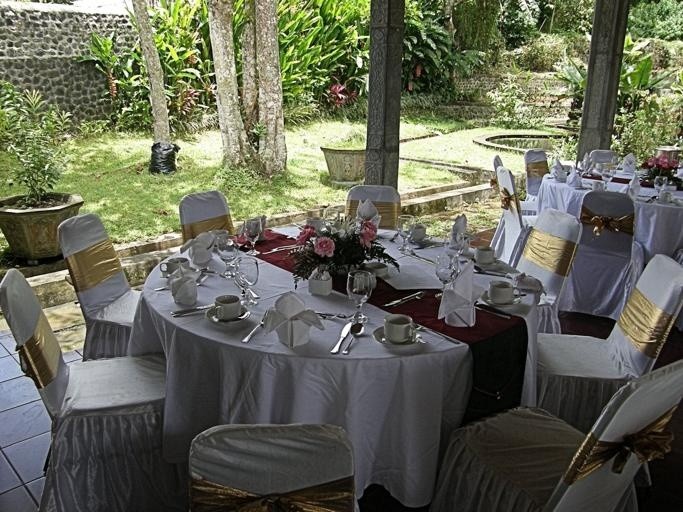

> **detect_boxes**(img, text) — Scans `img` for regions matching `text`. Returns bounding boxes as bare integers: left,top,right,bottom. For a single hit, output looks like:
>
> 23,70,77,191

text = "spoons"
472,266,511,279
343,324,364,354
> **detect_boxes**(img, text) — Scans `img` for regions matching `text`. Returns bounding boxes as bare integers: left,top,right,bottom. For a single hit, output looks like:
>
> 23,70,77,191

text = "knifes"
331,322,352,353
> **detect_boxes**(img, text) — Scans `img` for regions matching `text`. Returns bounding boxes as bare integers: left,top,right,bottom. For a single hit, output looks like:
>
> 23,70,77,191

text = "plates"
205,305,251,324
483,294,521,307
373,326,417,345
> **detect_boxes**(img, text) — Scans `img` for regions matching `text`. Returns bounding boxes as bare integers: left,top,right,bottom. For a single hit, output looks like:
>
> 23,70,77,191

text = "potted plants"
319,131,367,187
0,82,85,260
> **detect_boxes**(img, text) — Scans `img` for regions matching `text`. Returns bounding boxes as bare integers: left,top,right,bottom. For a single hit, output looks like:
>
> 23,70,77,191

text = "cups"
476,246,496,263
490,280,516,302
160,257,190,273
383,313,416,342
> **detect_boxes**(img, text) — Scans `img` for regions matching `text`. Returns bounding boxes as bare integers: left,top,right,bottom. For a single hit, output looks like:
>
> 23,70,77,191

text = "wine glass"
215,211,456,324
576,161,669,197
